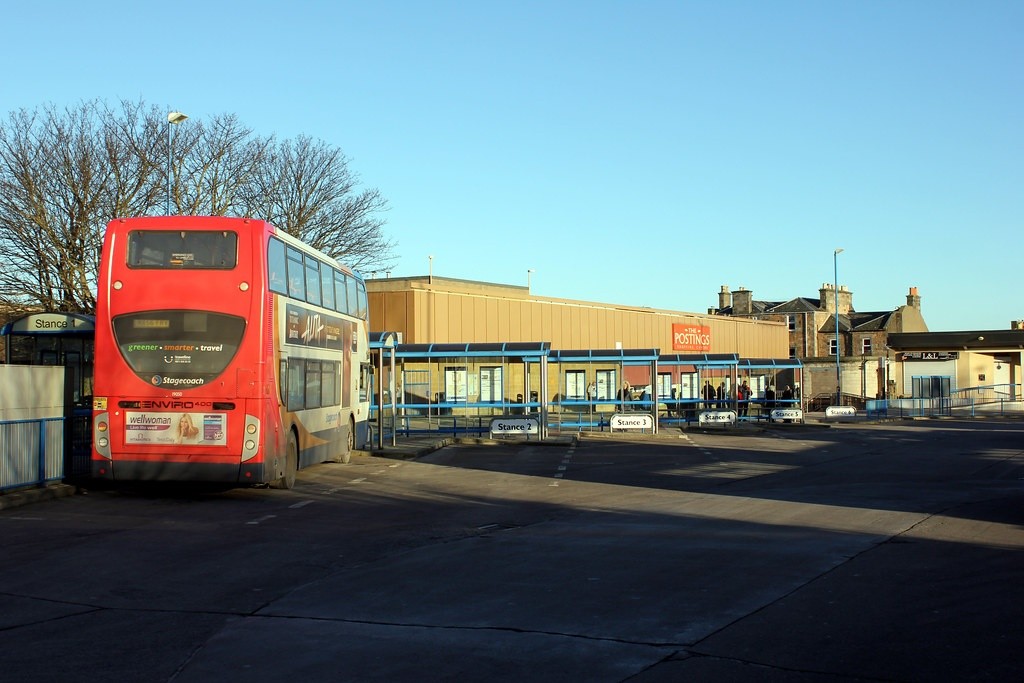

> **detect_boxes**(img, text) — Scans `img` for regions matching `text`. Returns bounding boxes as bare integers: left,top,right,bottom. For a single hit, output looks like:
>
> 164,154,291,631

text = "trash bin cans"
435,392,446,415
530,391,538,416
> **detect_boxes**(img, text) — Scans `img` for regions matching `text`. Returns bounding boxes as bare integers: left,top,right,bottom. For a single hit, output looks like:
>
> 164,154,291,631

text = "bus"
93,216,373,490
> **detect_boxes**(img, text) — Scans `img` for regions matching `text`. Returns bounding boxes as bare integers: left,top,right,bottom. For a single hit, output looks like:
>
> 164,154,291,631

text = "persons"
765,384,776,423
640,390,650,411
588,381,597,413
616,381,633,433
792,385,800,399
716,382,726,409
780,385,792,423
701,380,716,412
735,380,753,422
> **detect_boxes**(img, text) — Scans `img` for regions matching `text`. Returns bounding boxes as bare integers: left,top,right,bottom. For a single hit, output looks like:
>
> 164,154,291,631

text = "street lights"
165,113,187,215
834,248,845,405
